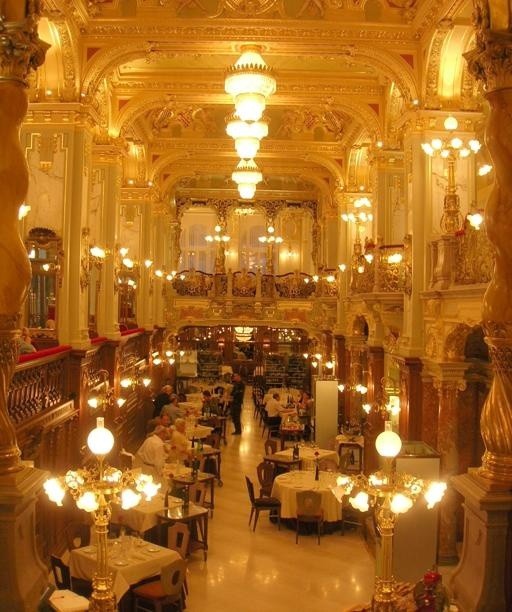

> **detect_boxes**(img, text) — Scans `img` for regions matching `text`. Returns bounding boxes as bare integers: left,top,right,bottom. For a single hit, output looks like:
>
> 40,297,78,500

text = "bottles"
293,444,299,459
182,485,189,509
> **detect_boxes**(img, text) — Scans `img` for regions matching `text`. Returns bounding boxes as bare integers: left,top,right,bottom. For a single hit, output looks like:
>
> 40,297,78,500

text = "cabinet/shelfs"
391,440,440,583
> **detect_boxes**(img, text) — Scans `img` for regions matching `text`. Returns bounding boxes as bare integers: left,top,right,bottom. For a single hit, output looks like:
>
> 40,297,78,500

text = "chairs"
243,475,281,532
252,375,381,546
50,375,236,612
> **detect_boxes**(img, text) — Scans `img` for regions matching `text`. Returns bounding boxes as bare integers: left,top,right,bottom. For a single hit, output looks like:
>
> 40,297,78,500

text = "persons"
265,393,293,438
300,392,313,441
202,391,220,416
135,385,192,499
230,373,244,435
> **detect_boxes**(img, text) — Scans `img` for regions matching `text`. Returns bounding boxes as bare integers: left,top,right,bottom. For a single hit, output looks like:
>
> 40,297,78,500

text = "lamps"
223,110,271,162
423,118,492,231
225,46,277,125
303,339,400,419
88,349,185,411
231,159,263,200
43,228,185,296
306,235,412,296
